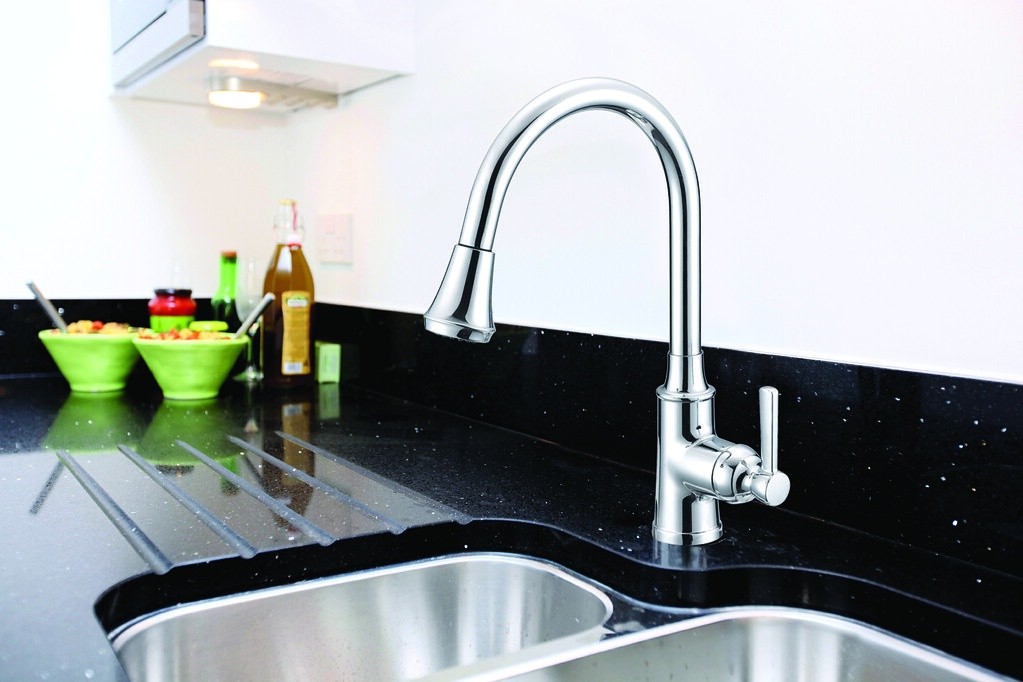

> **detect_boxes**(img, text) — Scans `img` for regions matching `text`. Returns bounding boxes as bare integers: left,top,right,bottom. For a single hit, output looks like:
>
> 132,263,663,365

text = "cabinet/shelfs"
108,0,410,121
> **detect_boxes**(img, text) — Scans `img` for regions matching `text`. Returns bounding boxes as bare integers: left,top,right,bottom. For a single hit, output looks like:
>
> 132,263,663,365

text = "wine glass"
235,254,264,382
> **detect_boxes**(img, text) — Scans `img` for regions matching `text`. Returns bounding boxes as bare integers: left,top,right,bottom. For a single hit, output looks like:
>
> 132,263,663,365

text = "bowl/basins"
131,331,250,401
38,326,142,394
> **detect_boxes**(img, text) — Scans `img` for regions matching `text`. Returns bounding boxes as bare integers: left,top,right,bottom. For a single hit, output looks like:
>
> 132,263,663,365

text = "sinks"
106,546,614,682
489,605,1023,682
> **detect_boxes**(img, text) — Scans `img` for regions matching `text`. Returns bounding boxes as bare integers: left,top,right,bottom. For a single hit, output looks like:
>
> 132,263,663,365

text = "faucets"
420,76,793,549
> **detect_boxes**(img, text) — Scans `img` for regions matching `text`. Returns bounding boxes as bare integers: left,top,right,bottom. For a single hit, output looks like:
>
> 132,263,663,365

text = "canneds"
149,288,195,332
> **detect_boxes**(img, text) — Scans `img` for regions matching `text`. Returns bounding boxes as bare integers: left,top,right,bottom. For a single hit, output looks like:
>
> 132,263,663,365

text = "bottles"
210,249,249,386
260,199,315,390
